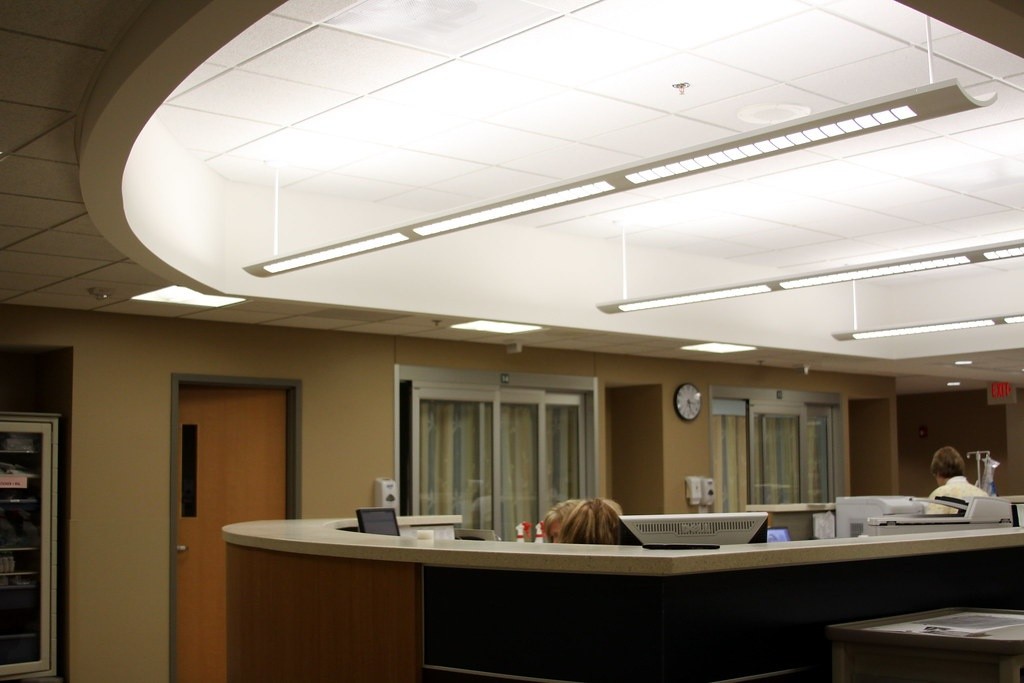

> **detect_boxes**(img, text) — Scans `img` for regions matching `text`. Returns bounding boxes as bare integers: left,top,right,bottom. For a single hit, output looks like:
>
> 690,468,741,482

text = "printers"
864,495,1013,536
836,497,928,540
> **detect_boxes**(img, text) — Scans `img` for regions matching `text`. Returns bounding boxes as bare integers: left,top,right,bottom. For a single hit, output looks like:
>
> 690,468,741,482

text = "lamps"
243,11,1000,284
601,210,1024,342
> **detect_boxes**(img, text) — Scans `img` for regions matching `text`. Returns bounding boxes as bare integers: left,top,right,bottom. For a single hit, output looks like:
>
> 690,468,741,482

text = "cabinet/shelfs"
0,413,66,681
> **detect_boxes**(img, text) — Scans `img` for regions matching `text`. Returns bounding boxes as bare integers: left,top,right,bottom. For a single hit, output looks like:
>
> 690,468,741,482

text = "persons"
542,499,620,545
927,446,988,513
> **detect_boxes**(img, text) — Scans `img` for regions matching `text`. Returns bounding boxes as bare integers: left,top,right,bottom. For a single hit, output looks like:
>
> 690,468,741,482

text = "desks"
744,495,1023,540
219,513,1024,683
828,604,1024,683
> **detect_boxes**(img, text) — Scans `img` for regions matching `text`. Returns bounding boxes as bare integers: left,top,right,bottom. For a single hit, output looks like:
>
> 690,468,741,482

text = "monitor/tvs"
618,511,792,545
356,508,400,537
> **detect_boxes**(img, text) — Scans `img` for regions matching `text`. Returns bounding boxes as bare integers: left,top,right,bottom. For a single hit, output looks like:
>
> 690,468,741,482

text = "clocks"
674,383,702,422
